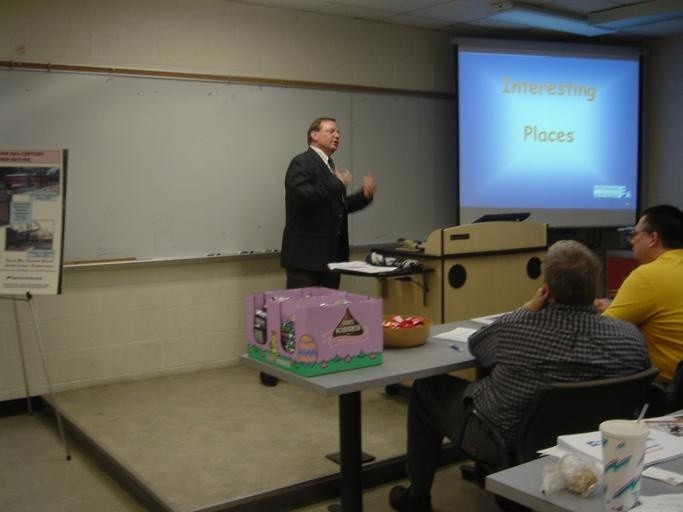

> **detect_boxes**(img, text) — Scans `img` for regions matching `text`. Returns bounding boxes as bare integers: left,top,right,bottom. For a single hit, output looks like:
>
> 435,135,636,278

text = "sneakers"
260,371,280,387
389,485,433,511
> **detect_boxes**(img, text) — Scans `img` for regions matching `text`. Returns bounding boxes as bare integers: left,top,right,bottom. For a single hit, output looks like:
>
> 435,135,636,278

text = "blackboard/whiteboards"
0,61,459,268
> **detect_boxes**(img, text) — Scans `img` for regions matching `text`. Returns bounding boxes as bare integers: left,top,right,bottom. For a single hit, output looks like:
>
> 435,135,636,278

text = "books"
557,427,682,469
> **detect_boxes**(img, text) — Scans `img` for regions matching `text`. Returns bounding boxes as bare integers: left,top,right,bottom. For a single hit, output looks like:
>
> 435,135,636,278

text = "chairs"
455,365,663,512
647,359,683,417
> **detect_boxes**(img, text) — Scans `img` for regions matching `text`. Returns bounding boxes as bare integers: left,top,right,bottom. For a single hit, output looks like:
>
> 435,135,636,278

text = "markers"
206,247,280,257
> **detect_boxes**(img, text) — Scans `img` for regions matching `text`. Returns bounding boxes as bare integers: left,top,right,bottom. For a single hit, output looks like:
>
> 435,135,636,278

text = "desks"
239,319,489,512
485,456,683,512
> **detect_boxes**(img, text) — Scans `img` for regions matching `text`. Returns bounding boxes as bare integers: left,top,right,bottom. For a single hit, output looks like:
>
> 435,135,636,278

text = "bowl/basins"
371,313,433,347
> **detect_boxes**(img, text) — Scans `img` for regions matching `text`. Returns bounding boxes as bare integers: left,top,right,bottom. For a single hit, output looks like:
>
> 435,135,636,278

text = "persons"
384,239,653,511
593,203,682,394
258,115,377,386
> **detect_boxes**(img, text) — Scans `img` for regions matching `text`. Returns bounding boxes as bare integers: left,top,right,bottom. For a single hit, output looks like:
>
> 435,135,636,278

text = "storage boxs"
246,287,384,378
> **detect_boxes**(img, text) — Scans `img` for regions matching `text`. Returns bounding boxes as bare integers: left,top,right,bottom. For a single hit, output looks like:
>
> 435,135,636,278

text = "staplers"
394,238,421,252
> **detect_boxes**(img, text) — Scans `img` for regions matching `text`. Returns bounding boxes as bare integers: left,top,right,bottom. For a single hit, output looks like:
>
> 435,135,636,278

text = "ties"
329,159,334,174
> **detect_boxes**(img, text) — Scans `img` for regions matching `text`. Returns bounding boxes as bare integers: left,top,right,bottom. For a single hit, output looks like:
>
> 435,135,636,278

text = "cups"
599,420,649,512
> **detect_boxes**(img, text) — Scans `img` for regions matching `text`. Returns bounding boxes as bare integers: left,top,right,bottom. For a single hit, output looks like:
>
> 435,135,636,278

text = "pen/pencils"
448,343,463,352
